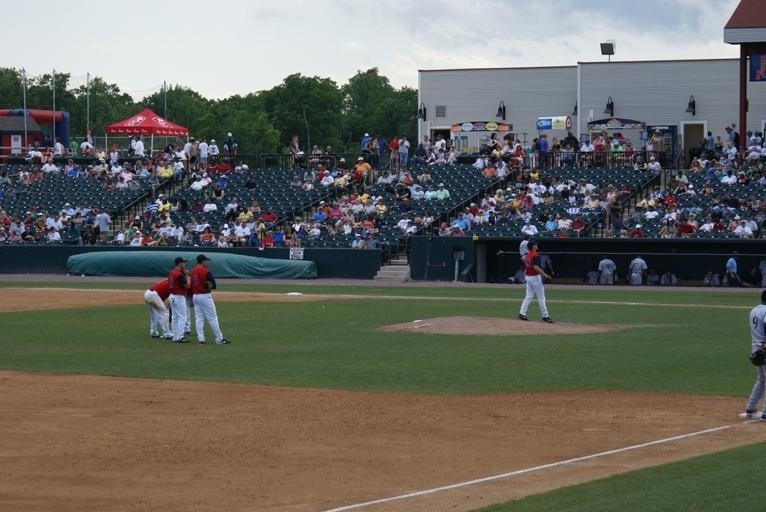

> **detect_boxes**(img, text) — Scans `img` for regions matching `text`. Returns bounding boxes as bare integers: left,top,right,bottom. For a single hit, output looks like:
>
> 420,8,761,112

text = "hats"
23,124,759,229
174,256,189,263
527,240,537,249
196,254,211,262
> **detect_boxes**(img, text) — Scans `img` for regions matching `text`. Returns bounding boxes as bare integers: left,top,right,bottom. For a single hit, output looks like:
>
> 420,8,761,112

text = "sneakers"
148,329,232,345
759,413,766,422
519,314,554,324
746,408,757,416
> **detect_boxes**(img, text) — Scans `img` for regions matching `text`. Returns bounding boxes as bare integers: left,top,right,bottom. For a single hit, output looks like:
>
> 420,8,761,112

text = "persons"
215,133,459,251
745,290,766,422
518,241,553,324
438,127,765,239
583,254,764,286
1,133,237,250
515,241,553,286
169,257,191,343
143,278,172,340
191,255,232,345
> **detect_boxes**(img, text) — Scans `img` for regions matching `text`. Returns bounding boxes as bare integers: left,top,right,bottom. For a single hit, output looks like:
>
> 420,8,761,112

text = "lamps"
496,100,506,120
600,43,614,62
572,100,577,115
604,96,614,117
416,102,427,121
685,94,695,116
